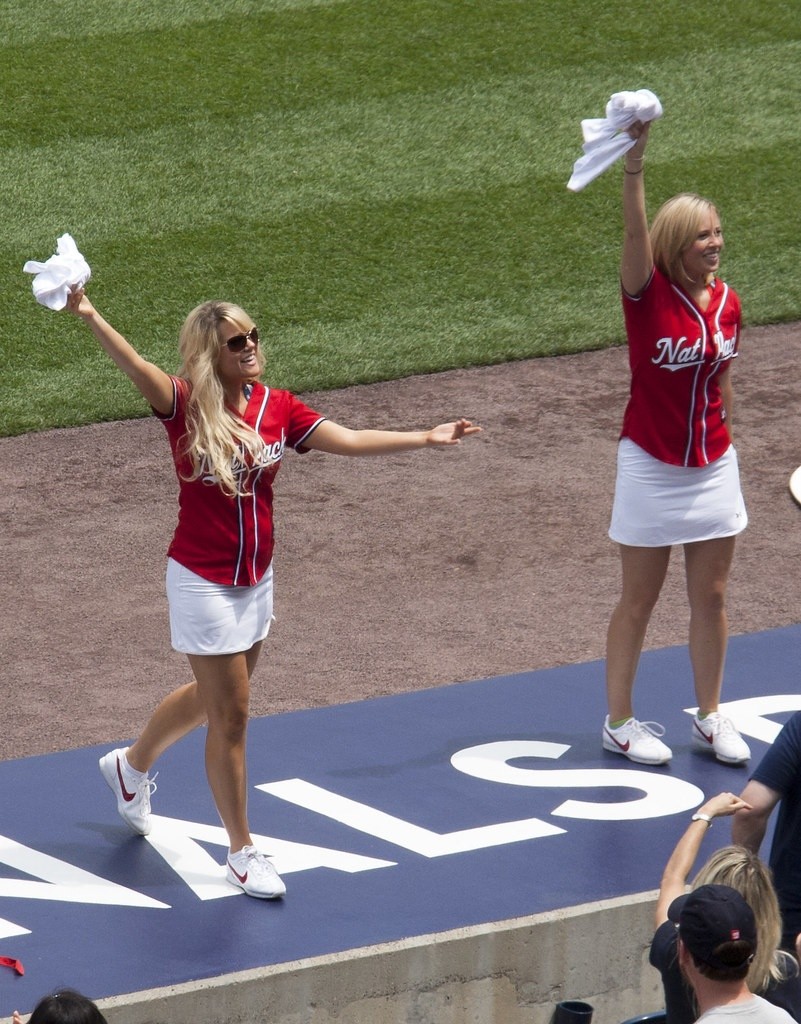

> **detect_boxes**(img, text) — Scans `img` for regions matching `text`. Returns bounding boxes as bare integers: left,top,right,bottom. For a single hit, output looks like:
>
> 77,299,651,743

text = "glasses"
221,327,258,352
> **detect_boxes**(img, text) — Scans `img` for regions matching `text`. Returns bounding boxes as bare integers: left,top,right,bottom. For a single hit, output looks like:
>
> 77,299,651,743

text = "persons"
65,281,481,898
667,885,800,1024
14,988,107,1024
649,791,801,1024
731,710,801,962
602,120,750,764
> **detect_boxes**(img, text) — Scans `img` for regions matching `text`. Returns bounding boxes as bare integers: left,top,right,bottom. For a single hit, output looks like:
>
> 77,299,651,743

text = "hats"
668,883,759,968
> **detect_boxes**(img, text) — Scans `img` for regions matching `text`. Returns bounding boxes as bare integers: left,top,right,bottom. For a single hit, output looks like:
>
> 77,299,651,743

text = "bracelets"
632,157,644,161
624,165,644,175
692,814,712,828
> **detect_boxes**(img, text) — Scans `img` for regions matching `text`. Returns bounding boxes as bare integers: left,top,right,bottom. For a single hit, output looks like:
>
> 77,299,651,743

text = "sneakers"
98,747,158,836
602,714,673,765
692,712,751,763
228,845,285,899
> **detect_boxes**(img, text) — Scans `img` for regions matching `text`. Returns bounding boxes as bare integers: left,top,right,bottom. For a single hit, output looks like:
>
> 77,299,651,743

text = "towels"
21,232,92,312
565,89,664,195
